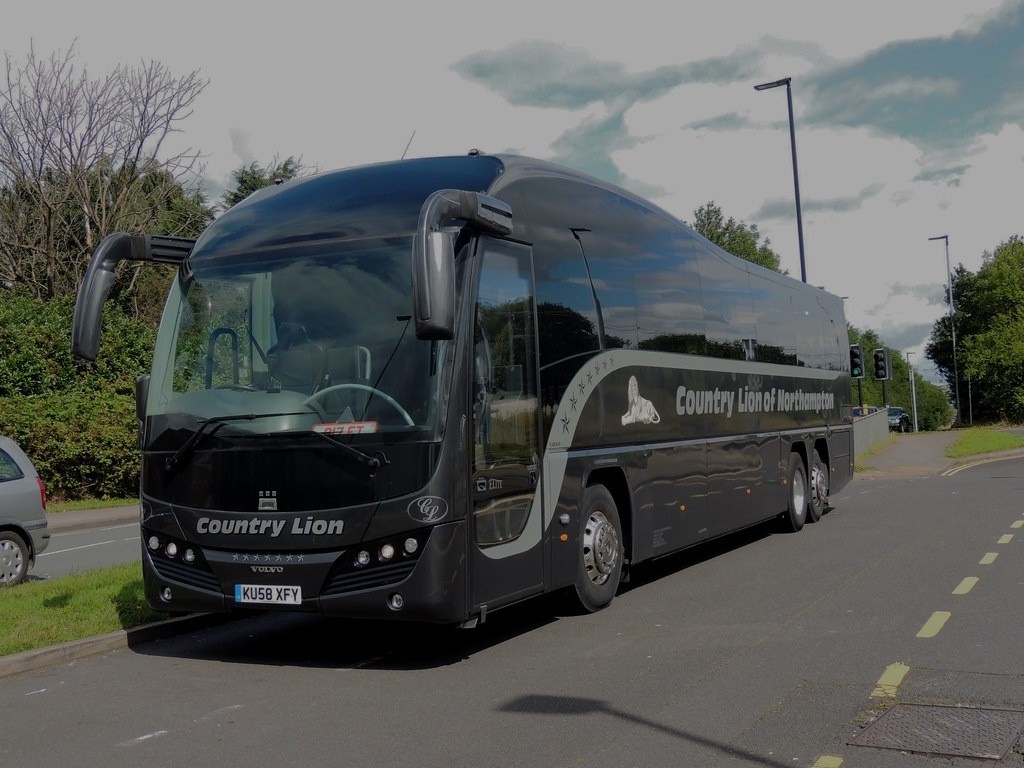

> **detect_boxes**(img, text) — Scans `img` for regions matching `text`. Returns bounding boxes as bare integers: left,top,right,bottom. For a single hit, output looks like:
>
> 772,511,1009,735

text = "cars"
852,406,878,423
886,406,911,433
0,434,52,588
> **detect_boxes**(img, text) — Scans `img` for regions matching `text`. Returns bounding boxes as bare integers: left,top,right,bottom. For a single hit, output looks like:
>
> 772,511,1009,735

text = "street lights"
753,76,807,283
907,351,916,368
928,234,961,428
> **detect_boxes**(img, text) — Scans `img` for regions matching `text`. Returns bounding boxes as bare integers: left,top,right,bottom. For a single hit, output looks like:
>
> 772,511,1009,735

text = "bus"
69,129,855,635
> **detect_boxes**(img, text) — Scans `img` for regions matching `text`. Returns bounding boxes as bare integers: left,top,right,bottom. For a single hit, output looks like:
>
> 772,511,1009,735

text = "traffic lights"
849,345,865,378
872,347,892,381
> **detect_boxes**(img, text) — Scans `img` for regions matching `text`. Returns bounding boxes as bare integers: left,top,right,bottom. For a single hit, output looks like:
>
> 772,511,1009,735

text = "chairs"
265,322,326,397
